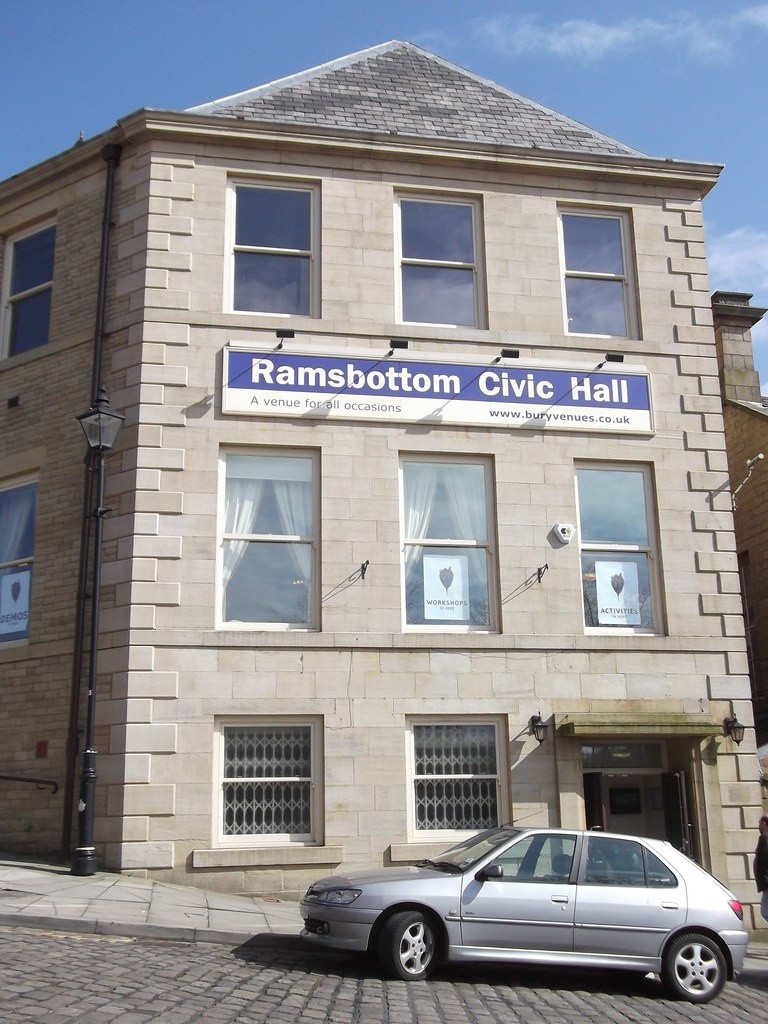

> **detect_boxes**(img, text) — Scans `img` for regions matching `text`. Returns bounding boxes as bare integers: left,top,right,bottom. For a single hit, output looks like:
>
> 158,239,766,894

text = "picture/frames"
609,786,642,814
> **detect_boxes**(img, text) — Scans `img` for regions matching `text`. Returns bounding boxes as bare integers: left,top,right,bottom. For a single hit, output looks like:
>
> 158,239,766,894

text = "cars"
298,828,747,1002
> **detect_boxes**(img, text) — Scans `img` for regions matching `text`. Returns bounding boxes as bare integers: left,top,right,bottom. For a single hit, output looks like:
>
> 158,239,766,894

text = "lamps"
273,328,626,371
609,745,632,758
531,711,549,746
725,713,745,746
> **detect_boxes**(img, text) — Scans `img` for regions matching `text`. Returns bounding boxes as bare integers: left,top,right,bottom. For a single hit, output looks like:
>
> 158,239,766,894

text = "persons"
752,814,768,922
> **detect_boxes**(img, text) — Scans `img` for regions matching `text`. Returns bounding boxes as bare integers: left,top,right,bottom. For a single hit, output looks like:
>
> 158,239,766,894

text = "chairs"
543,853,616,884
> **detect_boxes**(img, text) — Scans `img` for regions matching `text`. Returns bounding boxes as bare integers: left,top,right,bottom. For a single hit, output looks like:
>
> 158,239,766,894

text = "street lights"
80,401,119,850
